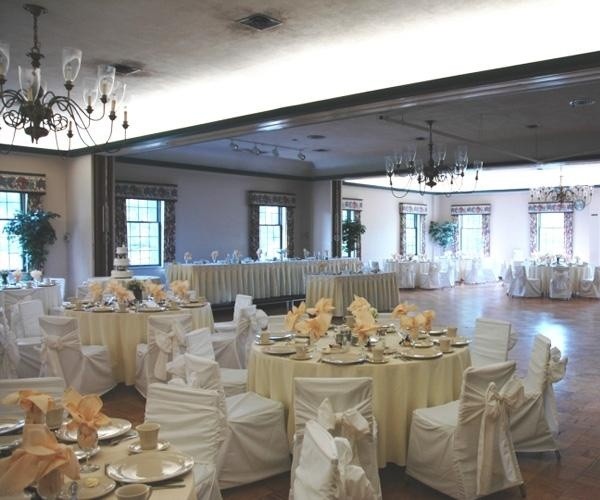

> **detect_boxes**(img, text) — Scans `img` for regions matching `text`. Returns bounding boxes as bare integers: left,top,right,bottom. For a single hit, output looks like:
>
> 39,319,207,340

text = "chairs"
18,299,46,338
404,359,528,499
549,267,573,301
397,263,416,289
211,306,258,369
0,307,44,379
418,262,441,289
135,314,193,399
143,381,227,499
576,266,600,299
440,263,455,288
506,265,541,298
37,315,117,397
288,377,383,499
186,327,249,397
470,316,511,367
508,334,567,462
168,353,291,491
213,293,252,334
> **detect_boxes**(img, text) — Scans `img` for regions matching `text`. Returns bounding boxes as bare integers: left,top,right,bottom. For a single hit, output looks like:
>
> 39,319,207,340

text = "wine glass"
76,424,99,473
37,473,63,500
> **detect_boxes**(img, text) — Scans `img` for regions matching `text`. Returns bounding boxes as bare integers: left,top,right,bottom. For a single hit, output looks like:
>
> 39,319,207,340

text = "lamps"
530,164,592,209
384,119,484,199
0,4,128,156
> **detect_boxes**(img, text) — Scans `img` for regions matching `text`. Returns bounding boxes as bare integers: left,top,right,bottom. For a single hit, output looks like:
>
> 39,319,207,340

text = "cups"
261,332,270,343
116,484,151,499
119,303,126,310
75,301,83,309
137,423,159,449
171,302,176,309
26,282,32,288
198,297,204,304
439,338,452,350
296,344,307,357
373,347,385,361
52,280,55,285
344,316,354,325
448,328,458,338
47,405,64,426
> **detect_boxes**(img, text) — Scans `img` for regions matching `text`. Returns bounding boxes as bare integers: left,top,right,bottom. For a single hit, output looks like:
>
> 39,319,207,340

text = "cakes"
110,247,133,279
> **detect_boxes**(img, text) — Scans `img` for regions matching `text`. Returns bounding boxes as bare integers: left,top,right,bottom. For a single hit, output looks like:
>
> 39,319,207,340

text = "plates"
181,305,203,308
69,444,99,460
57,416,132,442
58,475,115,500
6,287,21,289
256,331,295,339
130,439,170,451
0,419,24,434
50,417,72,430
401,348,442,358
320,353,365,365
433,337,472,345
420,328,447,334
413,339,433,347
263,345,296,354
92,306,114,312
38,284,54,287
138,306,165,312
106,452,194,483
